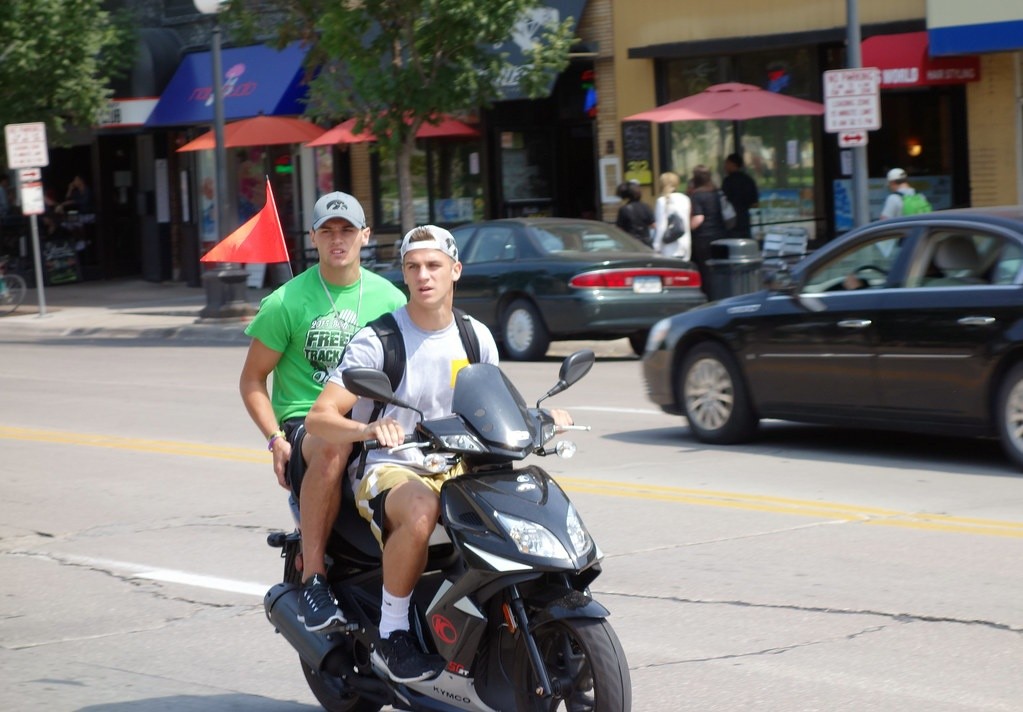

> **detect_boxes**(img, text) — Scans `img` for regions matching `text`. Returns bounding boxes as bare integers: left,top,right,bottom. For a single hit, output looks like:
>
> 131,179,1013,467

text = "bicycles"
0,256,26,315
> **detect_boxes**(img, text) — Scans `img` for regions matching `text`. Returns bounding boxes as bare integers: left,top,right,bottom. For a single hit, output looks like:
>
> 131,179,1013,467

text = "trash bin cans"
706,239,763,300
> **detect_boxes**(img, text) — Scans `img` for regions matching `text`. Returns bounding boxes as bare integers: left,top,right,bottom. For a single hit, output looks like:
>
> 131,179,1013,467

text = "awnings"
861,31,981,89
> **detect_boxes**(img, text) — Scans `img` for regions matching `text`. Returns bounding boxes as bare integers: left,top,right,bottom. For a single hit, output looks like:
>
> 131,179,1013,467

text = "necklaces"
317,263,364,345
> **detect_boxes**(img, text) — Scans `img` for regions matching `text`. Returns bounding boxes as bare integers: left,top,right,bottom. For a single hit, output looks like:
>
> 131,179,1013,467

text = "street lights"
195,0,254,324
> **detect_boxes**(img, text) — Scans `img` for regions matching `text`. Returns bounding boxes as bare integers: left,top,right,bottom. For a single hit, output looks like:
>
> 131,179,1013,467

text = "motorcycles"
263,349,634,712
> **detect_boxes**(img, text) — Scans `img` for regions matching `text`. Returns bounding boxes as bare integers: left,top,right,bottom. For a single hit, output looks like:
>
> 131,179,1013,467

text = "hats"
312,191,367,230
401,224,458,264
886,169,907,182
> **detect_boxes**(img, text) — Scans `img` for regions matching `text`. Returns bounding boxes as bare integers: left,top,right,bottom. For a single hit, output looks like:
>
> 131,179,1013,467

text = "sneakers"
369,628,435,683
297,574,347,633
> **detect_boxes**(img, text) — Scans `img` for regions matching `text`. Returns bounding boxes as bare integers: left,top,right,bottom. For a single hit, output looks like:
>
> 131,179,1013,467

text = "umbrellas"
304,107,478,152
621,82,824,172
174,115,327,198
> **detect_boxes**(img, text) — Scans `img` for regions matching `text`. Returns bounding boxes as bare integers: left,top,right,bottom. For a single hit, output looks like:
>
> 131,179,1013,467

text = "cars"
371,216,708,361
643,207,1023,472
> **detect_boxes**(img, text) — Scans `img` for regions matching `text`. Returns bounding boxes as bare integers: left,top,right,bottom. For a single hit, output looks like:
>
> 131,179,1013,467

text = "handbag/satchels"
714,185,736,220
661,194,686,244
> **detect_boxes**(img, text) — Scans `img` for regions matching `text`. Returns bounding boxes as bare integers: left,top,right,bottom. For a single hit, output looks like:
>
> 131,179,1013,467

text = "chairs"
927,235,990,286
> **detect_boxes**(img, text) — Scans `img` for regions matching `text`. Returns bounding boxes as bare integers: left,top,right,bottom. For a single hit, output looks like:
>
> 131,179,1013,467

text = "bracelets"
267,430,286,453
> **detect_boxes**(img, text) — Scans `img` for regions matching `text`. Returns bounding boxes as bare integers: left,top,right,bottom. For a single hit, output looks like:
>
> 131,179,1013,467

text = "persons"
879,168,917,221
616,150,761,263
305,223,576,685
236,189,408,634
844,237,904,290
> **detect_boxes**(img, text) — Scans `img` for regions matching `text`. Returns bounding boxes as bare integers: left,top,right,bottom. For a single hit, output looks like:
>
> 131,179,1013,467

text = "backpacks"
890,188,932,218
